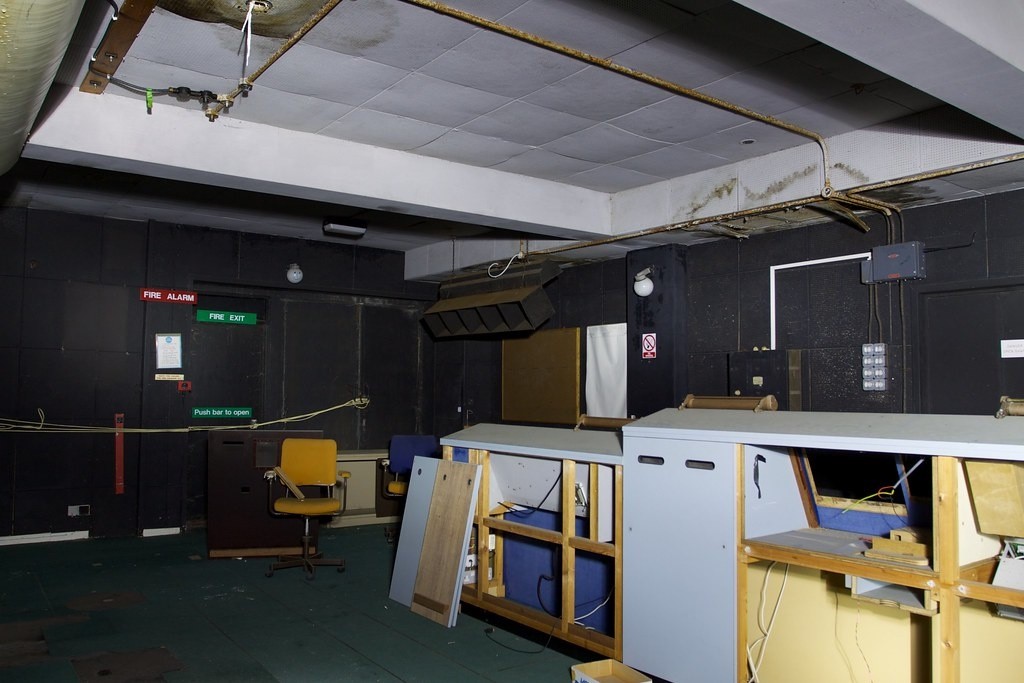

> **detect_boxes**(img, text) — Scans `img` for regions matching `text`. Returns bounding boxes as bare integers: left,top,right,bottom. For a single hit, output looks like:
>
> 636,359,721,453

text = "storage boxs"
571,661,655,683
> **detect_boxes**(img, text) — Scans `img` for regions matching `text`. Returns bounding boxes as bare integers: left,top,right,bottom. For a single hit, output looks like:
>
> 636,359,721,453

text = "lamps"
633,265,664,298
323,217,368,234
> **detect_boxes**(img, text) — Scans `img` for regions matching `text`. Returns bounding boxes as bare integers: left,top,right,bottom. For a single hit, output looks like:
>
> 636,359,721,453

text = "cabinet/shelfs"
440,421,621,658
622,408,1023,683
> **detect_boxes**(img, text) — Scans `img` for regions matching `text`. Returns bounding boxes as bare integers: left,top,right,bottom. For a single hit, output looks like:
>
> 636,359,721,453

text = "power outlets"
863,342,888,392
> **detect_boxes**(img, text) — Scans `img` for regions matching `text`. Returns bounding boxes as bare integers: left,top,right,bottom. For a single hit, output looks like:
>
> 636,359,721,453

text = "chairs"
381,434,441,544
264,437,352,580
